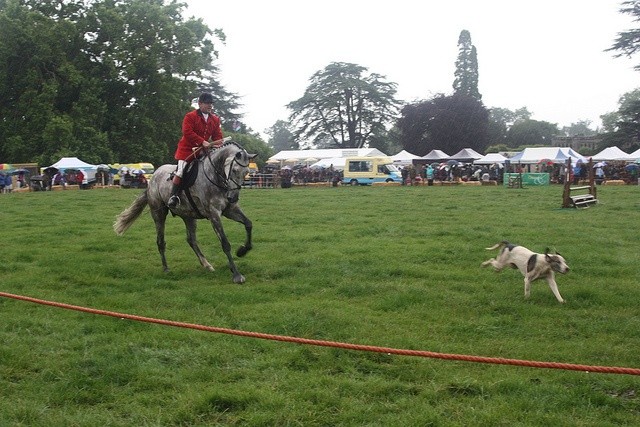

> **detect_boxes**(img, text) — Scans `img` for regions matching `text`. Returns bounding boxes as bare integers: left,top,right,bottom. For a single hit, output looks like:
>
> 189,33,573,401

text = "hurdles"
562,156,599,210
507,160,522,189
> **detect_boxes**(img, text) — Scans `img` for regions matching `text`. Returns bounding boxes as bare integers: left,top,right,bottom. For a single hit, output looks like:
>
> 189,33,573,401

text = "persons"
506,164,514,172
0,172,6,193
516,164,523,173
168,93,223,208
135,174,147,180
118,171,125,186
19,171,30,187
3,172,13,196
535,160,582,186
402,161,503,186
595,168,605,184
55,169,84,188
250,164,343,187
126,171,131,179
47,169,52,190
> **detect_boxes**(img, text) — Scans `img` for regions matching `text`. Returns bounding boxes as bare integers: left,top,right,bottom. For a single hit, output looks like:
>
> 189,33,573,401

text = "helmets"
199,93,214,103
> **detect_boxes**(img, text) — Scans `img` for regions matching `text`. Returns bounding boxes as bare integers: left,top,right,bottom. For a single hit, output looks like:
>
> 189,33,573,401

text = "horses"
113,141,260,286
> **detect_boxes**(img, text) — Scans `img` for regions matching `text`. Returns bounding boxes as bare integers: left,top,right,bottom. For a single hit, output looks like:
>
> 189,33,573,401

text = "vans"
342,157,404,188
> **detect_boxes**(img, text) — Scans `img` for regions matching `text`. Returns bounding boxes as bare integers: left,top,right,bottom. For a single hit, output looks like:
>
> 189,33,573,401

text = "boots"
168,175,183,206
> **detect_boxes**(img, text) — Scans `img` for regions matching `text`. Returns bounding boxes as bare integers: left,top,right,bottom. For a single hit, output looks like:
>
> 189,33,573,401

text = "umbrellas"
286,158,300,162
266,158,281,163
625,164,638,170
11,168,31,174
593,162,607,168
117,166,131,172
131,168,146,175
30,175,44,181
0,163,13,170
42,167,59,173
305,157,318,162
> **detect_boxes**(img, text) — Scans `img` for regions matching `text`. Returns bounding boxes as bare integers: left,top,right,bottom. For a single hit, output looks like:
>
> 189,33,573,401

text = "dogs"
481,240,571,305
